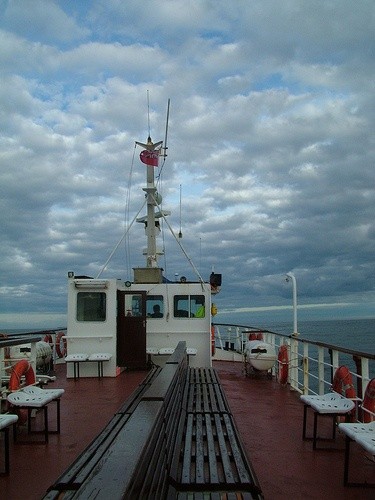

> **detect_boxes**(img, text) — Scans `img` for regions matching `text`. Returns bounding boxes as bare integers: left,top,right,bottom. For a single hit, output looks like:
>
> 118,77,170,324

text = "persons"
152,304,163,318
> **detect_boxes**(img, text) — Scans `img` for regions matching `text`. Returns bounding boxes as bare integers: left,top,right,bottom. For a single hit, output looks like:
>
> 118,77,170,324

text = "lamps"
180,276,186,283
125,280,132,287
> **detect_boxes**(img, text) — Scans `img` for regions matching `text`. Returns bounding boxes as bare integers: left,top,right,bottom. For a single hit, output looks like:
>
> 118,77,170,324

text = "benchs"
114,364,179,413
337,420,375,489
138,340,187,385
71,401,267,500
6,385,65,446
164,341,224,385
113,348,186,415
299,392,356,453
0,413,18,477
64,353,112,385
40,400,165,500
159,347,198,365
140,363,230,413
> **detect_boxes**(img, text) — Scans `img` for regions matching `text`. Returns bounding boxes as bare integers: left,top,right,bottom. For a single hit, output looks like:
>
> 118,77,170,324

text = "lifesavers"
45,336,50,344
56,332,67,357
362,378,375,423
211,326,215,356
333,366,357,423
9,359,36,425
249,333,263,341
277,345,288,383
